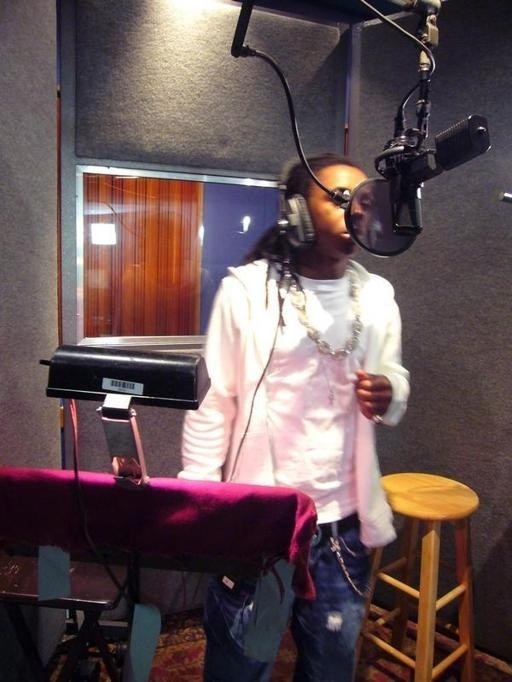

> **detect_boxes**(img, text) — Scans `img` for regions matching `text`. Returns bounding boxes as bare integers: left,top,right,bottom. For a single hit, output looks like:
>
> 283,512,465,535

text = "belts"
311,512,359,547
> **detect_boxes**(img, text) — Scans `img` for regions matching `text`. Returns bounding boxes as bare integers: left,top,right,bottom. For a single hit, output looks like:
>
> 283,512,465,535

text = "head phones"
275,175,317,255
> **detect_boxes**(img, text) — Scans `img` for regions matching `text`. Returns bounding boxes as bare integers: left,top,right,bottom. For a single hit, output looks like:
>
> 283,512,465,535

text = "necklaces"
284,257,362,360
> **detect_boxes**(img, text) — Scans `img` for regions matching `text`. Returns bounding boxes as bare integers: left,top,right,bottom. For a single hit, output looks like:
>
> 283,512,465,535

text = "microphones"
343,114,493,258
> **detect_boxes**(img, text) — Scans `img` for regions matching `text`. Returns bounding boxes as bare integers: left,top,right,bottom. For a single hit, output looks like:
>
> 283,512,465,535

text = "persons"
177,155,410,682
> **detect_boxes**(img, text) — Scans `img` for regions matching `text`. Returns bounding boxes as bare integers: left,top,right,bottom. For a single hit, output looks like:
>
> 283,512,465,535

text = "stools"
1,550,132,682
359,473,480,682
